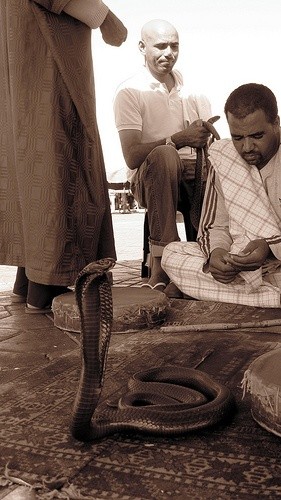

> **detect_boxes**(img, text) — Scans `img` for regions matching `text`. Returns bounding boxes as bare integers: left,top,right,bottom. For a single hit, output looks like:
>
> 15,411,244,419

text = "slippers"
11,292,25,303
22,302,52,315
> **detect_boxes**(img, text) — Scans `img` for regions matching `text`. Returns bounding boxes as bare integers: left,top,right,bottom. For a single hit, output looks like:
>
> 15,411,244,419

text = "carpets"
1,299,281,500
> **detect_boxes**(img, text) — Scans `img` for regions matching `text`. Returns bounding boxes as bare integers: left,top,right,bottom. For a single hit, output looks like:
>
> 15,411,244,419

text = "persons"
158,83,281,308
112,17,223,292
1,0,130,316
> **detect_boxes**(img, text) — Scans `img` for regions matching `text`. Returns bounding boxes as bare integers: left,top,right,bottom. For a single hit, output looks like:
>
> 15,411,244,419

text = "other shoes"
146,257,168,290
163,280,185,298
